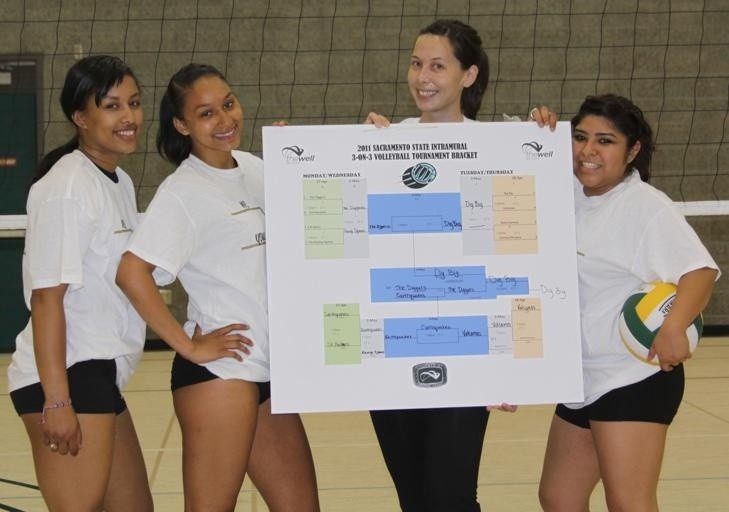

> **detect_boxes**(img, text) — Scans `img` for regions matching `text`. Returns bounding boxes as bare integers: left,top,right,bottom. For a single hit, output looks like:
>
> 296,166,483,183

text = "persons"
113,63,320,511
361,17,517,512
523,94,720,512
8,53,158,510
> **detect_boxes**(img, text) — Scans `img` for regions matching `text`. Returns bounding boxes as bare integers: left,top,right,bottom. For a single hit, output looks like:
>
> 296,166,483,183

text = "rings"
530,107,538,118
46,441,54,447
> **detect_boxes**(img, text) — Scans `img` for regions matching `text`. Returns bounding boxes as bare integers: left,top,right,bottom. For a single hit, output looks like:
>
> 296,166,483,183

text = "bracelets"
38,399,76,411
